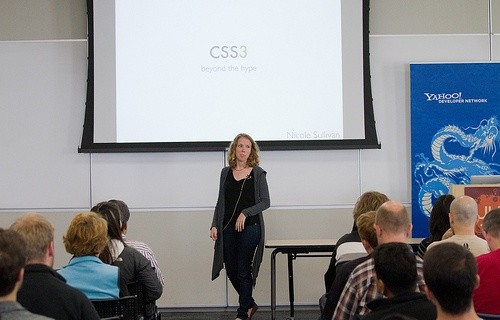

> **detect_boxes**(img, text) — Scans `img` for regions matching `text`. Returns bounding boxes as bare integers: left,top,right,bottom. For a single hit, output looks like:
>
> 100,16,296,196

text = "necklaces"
235,165,247,171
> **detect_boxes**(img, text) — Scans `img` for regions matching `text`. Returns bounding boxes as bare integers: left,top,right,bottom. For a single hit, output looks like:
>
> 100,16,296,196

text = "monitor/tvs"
471,175,500,184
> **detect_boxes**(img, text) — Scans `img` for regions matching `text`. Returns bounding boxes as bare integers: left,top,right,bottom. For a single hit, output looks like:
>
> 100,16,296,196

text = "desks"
263,239,341,320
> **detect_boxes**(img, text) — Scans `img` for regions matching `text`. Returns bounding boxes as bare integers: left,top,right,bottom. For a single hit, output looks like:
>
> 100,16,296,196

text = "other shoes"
247,304,258,320
234,314,243,320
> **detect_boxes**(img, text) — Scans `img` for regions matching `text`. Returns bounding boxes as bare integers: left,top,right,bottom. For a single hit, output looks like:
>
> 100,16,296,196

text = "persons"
317,192,500,320
0,200,165,320
210,133,270,320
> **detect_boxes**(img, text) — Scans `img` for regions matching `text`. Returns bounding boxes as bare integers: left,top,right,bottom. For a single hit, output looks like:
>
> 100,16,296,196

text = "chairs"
86,279,162,320
